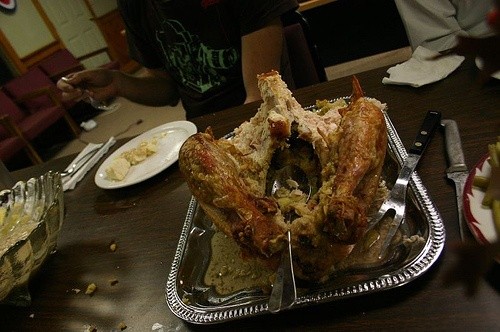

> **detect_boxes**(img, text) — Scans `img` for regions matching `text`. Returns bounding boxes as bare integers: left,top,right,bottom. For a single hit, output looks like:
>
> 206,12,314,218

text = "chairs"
0,47,120,166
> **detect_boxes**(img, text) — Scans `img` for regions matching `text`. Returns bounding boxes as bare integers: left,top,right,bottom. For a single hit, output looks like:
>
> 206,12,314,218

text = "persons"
393,1,500,55
56,0,328,121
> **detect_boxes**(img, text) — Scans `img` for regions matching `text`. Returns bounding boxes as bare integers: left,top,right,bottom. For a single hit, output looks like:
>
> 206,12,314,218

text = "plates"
463,153,500,263
94,120,197,190
0,170,64,301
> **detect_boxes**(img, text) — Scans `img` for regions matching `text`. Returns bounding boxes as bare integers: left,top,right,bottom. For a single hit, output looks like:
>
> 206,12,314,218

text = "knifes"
441,120,470,242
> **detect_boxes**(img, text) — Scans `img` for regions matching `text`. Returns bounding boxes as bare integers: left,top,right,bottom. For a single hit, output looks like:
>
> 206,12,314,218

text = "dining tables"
0,48,500,332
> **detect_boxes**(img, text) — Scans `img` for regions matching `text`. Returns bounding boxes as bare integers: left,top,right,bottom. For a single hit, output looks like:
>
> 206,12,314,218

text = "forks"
363,111,441,260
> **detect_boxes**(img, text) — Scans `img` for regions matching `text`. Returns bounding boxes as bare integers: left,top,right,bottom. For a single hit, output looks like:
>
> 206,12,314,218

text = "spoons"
271,164,312,309
61,77,114,110
56,148,98,177
